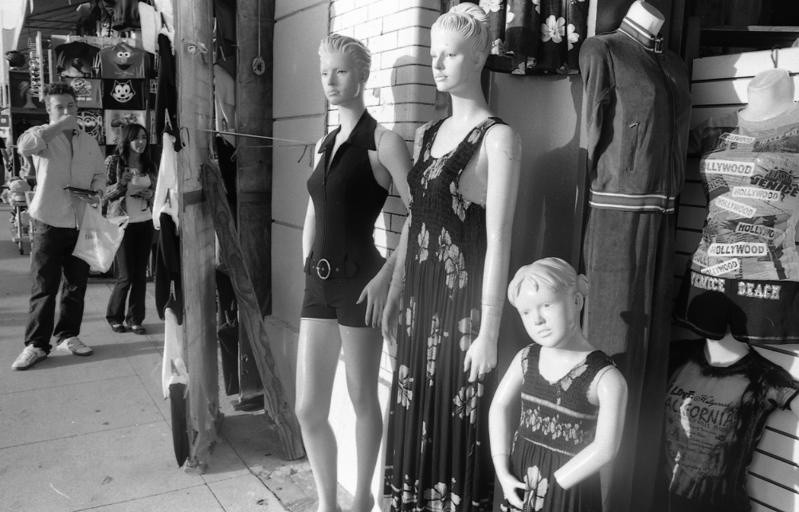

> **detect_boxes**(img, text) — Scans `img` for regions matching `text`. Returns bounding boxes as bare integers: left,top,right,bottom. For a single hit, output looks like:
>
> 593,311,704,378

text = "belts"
304,257,358,280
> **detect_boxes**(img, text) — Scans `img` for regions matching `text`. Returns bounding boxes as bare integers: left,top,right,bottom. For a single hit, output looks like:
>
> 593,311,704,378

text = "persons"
576,3,693,512
383,2,524,511
688,70,798,344
10,82,108,370
655,319,798,509
289,32,415,512
97,124,157,334
487,256,629,511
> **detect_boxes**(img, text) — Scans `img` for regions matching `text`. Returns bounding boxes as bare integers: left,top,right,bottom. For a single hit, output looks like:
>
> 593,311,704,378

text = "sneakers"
12,343,47,369
126,320,144,335
56,336,92,356
106,317,126,332
